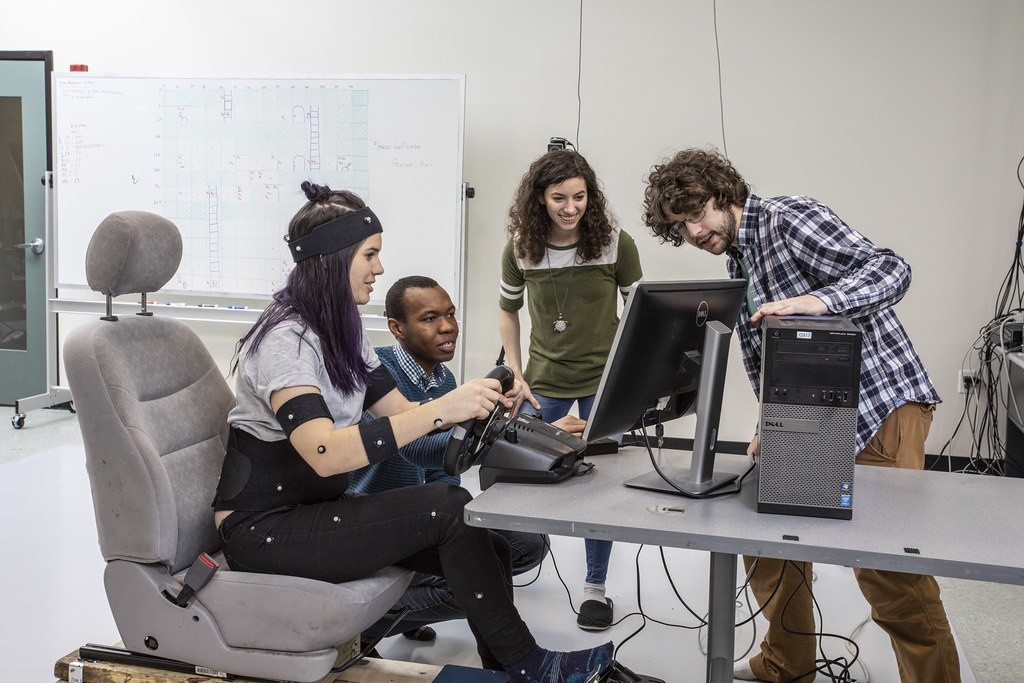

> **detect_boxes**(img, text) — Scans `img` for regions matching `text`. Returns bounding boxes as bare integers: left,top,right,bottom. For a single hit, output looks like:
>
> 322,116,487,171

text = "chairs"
63,210,416,682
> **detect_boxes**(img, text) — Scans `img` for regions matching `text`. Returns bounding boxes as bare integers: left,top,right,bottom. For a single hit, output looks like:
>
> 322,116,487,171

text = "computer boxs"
756,314,864,520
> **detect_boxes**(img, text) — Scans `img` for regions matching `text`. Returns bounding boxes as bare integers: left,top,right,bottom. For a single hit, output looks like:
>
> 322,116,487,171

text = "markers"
167,303,187,307
227,305,251,314
139,301,159,305
198,303,219,309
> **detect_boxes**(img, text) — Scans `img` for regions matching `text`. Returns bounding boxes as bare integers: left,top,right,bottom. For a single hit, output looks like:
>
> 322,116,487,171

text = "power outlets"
957,369,979,394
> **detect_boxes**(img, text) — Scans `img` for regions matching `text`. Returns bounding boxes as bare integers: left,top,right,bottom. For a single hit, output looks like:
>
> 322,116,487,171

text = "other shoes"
402,626,437,642
732,657,761,680
365,648,384,659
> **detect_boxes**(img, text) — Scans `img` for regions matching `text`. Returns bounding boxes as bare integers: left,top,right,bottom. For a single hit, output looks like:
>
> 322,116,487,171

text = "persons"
347,275,588,659
209,182,614,683
640,149,962,683
499,151,643,630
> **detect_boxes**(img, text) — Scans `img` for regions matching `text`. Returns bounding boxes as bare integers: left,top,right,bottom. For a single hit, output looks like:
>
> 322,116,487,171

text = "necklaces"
546,247,578,332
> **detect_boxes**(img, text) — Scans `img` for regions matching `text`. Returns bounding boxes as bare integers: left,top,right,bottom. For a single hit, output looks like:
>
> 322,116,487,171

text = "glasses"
669,194,714,238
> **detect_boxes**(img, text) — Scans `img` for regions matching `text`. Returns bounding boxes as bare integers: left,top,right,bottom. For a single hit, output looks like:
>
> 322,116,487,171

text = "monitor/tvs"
581,279,751,498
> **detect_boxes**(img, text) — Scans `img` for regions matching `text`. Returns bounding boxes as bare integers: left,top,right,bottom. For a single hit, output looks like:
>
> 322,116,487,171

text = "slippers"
577,596,613,632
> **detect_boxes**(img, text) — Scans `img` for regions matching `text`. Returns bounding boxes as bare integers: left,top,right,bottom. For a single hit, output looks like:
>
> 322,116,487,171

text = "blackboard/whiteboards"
52,68,468,309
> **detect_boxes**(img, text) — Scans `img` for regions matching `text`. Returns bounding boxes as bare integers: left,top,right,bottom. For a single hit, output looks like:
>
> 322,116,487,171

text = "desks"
462,445,1024,683
